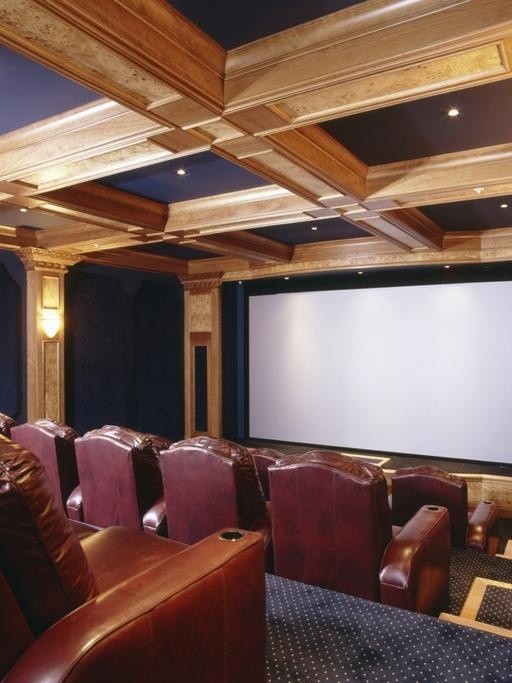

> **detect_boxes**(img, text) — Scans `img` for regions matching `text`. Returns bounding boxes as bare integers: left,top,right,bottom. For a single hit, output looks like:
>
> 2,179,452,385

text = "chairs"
9,420,84,523
390,464,498,550
75,425,167,539
265,448,453,615
1,433,269,682
157,437,271,571
